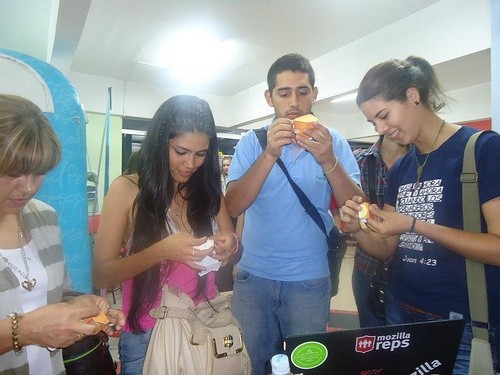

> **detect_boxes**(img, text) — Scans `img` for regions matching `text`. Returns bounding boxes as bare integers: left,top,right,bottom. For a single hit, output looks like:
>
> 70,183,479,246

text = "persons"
222,156,233,188
220,53,366,375
92,94,244,375
340,54,500,375
352,135,417,328
0,93,127,375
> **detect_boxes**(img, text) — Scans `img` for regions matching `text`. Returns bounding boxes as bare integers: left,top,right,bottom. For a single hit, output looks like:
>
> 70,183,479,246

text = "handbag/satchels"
143,283,251,375
328,226,347,297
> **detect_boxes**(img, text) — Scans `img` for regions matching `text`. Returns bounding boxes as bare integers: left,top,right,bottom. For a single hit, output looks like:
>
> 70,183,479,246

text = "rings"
224,250,227,254
75,333,82,340
192,241,194,246
193,249,194,256
102,304,112,308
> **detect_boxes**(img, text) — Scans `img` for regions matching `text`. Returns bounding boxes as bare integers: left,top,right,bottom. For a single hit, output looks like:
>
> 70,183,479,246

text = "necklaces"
0,213,37,292
173,202,185,216
413,120,445,187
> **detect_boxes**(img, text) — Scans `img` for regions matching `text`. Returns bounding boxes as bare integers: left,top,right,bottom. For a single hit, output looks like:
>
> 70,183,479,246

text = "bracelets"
322,157,338,174
8,312,22,352
231,233,240,254
410,218,416,233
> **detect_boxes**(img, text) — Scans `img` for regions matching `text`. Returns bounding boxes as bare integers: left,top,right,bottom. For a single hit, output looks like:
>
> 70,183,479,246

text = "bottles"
270,354,293,375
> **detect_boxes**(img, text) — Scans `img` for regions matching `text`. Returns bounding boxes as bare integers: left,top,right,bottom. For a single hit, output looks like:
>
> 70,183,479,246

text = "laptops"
282,318,468,375
